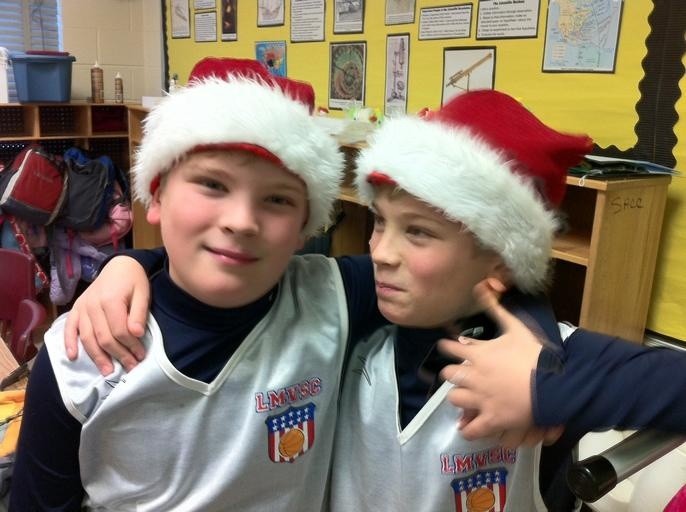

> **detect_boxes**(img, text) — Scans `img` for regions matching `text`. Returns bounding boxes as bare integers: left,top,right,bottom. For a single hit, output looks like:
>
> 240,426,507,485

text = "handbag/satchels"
0,145,132,247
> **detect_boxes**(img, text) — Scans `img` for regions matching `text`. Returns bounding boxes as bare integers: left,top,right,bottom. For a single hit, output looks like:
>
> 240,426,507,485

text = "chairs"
6,298,48,369
0,247,39,366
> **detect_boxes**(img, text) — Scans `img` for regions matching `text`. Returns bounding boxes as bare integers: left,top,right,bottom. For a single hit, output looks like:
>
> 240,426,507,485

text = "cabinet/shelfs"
543,162,673,349
122,104,164,254
324,129,371,259
0,96,143,146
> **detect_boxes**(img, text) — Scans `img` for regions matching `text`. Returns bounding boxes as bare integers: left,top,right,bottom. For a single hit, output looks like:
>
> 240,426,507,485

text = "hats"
357,90,593,294
134,59,344,240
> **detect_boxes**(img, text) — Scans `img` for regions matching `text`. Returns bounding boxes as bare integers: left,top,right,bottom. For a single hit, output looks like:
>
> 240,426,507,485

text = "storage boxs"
6,49,77,103
0,57,11,105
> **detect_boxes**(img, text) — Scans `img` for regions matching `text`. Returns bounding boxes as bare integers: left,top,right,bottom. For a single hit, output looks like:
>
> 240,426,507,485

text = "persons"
63,89,686,512
6,57,568,511
